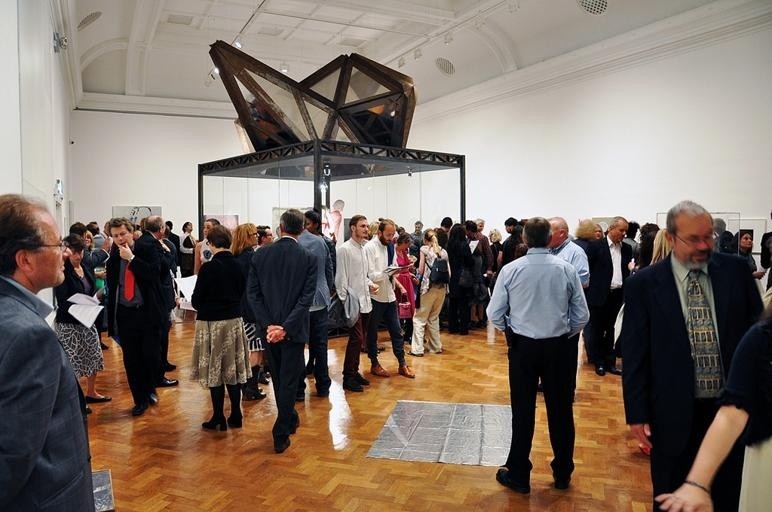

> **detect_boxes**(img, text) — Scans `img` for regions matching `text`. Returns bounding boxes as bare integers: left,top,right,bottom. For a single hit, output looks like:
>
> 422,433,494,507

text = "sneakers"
316,389,332,396
358,340,445,357
296,394,305,402
227,414,243,429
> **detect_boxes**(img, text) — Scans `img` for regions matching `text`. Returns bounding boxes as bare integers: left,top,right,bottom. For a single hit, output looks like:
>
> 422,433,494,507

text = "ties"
124,260,135,303
687,271,726,397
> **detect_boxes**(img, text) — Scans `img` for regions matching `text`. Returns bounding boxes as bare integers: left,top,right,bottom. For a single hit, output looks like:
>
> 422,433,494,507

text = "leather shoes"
154,376,179,387
273,418,300,455
131,401,150,416
370,363,391,377
496,466,534,494
355,372,370,386
551,473,572,488
596,363,606,376
85,395,111,404
341,377,364,393
167,362,177,371
85,404,92,415
397,364,415,377
150,391,159,406
606,365,623,375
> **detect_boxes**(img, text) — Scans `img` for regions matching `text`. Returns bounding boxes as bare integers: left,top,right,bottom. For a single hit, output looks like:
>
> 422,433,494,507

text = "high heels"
201,411,228,431
244,369,273,401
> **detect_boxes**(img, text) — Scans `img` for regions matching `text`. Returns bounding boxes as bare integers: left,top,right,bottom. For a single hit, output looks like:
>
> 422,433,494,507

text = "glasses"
32,238,68,252
249,232,258,236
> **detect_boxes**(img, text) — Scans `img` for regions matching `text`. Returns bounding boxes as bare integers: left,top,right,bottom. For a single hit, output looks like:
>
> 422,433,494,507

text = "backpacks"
429,257,451,285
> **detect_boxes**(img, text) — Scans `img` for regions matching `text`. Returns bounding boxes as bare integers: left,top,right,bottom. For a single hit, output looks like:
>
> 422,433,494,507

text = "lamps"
395,0,521,68
52,31,69,54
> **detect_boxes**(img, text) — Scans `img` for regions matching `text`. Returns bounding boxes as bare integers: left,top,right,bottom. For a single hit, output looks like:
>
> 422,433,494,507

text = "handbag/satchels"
396,293,414,320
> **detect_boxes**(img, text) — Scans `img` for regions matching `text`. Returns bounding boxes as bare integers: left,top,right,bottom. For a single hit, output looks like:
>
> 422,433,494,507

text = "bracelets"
684,480,712,495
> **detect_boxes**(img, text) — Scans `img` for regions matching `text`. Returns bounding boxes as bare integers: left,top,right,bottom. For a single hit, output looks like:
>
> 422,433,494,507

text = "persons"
655,298,771,512
708,218,771,292
622,199,767,511
0,194,95,512
57,209,527,455
486,218,590,493
549,217,673,377
